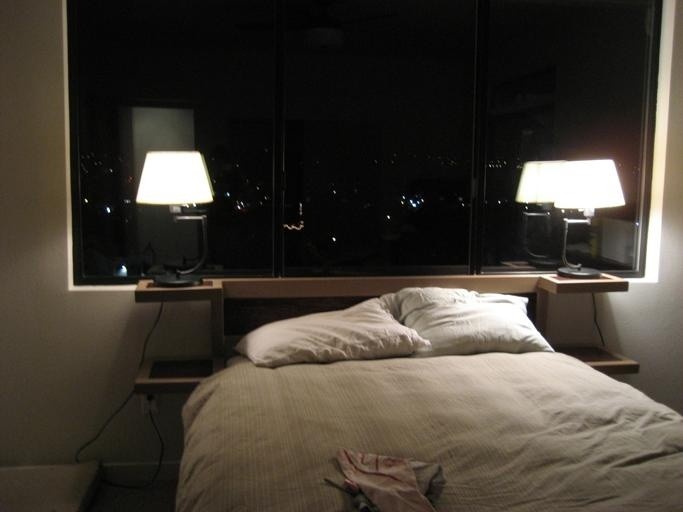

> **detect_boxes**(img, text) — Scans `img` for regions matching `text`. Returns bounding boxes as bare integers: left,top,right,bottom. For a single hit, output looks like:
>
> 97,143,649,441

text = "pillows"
381,287,555,358
235,298,430,368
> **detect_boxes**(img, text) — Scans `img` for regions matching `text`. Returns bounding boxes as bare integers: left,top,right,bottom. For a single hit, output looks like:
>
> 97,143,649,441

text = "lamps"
554,159,626,279
515,160,565,269
136,149,214,286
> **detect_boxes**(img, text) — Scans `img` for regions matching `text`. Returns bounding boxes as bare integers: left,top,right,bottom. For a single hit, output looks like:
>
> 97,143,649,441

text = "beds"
135,273,683,512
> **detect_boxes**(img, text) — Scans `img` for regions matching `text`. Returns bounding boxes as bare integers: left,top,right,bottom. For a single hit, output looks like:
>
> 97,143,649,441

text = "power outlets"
142,393,160,416
